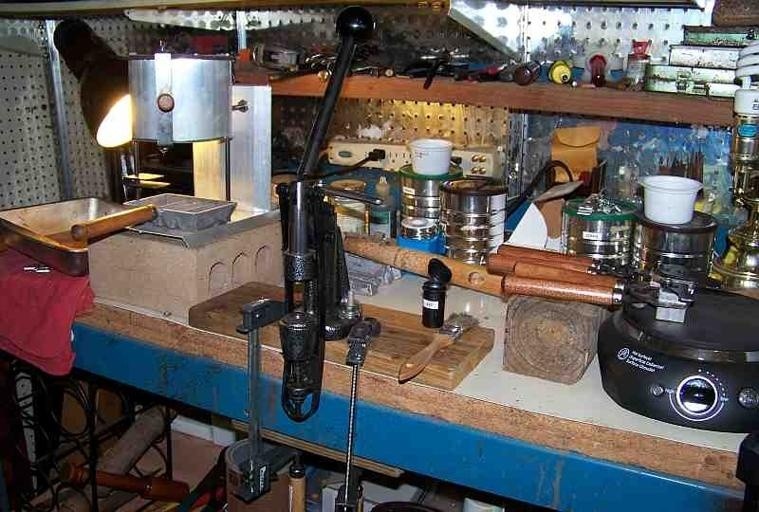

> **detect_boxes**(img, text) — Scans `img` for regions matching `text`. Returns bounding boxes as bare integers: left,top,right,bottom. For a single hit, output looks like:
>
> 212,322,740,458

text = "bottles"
421,279,448,330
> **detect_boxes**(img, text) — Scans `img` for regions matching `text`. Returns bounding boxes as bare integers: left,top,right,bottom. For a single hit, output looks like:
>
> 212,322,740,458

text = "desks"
1,251,756,509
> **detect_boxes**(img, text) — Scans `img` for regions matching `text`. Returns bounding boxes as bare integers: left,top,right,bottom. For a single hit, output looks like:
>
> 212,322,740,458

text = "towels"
3,243,93,380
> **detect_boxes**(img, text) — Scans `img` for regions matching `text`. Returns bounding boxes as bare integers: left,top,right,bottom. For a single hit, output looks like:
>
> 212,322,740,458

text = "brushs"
398,310,481,382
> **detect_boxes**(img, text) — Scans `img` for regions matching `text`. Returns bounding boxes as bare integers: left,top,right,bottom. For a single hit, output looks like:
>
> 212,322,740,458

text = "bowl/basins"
407,138,455,178
635,175,703,227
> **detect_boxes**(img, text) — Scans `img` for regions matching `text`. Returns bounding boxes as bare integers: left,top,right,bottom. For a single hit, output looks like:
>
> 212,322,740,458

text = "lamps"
52,16,134,149
